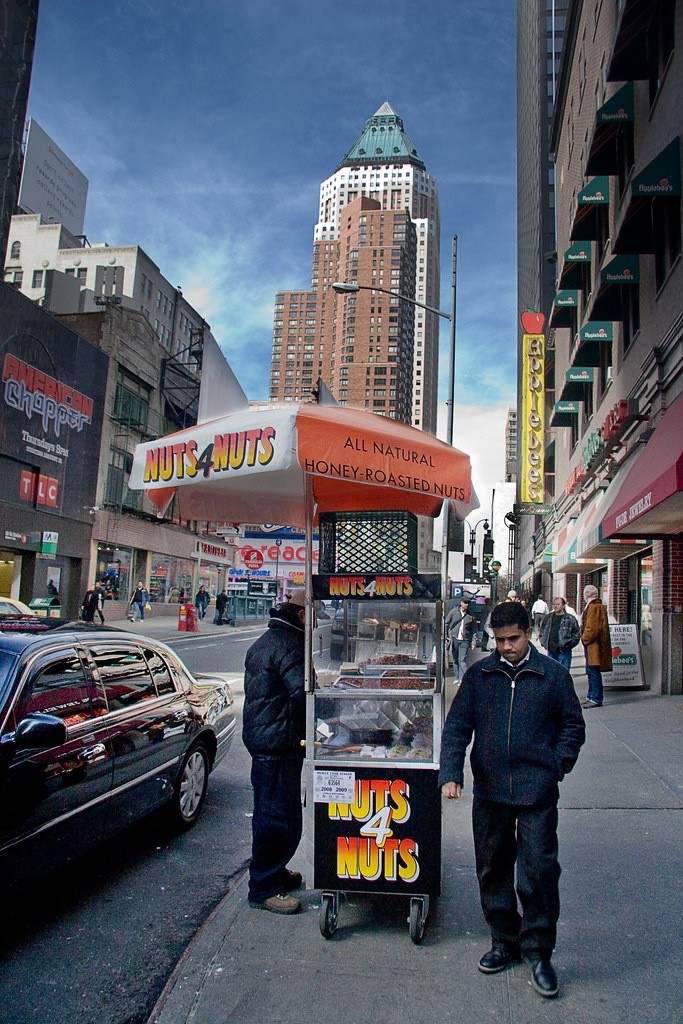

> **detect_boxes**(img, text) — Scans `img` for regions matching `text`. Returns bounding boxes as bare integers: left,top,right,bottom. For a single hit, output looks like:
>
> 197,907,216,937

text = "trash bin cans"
29,597,62,619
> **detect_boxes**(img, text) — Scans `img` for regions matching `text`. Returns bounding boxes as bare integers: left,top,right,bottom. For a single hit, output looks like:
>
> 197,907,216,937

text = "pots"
339,721,392,744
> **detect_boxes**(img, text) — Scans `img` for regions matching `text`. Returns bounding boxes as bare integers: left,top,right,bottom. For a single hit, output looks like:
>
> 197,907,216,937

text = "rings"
450,794,454,797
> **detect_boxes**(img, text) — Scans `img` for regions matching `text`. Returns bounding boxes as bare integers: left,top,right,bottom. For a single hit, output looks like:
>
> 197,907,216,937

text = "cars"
0,597,34,615
0,614,237,900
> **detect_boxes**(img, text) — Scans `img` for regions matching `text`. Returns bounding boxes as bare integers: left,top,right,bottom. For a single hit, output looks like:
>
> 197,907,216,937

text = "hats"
461,596,470,602
285,588,332,621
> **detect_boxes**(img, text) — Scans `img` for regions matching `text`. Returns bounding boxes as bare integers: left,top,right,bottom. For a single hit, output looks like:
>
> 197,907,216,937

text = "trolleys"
306,572,443,945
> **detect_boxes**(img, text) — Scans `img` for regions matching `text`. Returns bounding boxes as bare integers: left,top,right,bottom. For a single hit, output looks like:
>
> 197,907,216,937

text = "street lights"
331,282,458,694
464,518,490,573
491,561,502,605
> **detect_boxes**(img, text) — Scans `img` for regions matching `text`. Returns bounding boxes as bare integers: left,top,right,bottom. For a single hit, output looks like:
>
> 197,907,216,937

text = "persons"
195,586,210,621
47,579,58,595
216,589,228,625
578,585,613,709
242,591,334,914
81,580,107,625
445,596,477,687
170,586,184,604
439,602,586,996
128,580,149,623
474,590,580,673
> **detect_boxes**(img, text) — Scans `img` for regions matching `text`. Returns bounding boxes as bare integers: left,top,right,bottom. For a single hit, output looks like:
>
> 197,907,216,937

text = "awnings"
548,0,683,428
532,391,683,573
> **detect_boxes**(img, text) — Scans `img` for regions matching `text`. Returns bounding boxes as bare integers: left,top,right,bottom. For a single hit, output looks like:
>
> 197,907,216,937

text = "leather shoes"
580,699,597,708
478,949,513,973
523,955,559,997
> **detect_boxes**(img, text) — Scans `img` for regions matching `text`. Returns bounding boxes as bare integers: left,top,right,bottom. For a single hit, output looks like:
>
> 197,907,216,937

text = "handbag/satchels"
145,603,152,612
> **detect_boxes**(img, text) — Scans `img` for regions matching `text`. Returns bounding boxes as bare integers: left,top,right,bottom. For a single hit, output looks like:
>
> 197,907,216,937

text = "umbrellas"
127,403,481,692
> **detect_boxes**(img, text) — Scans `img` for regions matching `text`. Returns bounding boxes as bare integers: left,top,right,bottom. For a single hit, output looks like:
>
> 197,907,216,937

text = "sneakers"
140,620,144,623
249,891,301,914
284,868,303,886
453,680,462,686
131,617,135,623
